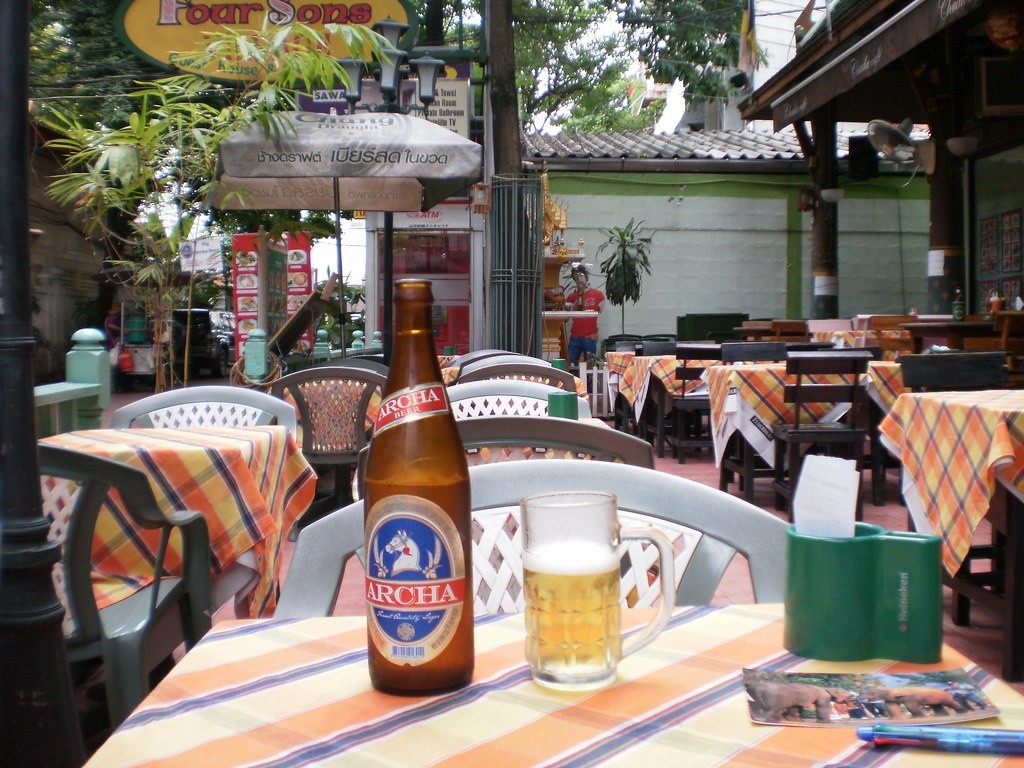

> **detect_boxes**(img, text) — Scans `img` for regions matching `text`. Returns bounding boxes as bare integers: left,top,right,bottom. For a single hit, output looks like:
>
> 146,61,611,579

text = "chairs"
38,312,1024,742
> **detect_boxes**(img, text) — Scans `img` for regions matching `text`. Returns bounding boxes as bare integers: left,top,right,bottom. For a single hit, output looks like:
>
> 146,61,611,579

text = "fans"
866,117,936,175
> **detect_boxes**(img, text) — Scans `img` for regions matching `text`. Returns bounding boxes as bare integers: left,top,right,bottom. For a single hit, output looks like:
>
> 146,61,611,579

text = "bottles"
363,278,475,698
953,287,967,323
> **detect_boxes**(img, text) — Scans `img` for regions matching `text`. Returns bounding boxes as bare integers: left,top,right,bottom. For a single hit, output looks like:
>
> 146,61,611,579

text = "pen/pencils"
856,722,1024,755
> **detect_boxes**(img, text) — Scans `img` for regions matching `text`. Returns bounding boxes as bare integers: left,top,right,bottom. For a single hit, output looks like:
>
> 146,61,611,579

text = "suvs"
172,308,231,379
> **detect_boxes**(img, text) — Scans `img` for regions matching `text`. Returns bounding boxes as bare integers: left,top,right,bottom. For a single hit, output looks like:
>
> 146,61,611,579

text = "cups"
519,491,622,691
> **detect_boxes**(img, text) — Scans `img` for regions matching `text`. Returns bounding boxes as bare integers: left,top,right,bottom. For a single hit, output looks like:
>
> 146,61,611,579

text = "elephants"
743,670,984,722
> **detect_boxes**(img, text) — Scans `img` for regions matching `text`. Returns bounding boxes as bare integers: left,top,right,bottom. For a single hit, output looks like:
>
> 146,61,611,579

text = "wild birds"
794,25,805,41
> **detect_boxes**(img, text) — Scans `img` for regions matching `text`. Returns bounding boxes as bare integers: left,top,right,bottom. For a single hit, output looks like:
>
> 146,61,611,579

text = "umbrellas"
211,107,482,359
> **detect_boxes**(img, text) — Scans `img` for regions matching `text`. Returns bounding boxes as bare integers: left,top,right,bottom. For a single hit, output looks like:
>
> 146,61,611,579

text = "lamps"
947,137,980,317
730,72,749,96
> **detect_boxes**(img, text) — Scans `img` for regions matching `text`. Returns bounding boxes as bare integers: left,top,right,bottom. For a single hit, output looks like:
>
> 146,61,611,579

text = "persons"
104,304,127,392
832,677,988,721
564,266,606,364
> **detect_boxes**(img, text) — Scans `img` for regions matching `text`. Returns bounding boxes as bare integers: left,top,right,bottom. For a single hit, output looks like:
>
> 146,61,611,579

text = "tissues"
783,453,947,663
929,345,961,352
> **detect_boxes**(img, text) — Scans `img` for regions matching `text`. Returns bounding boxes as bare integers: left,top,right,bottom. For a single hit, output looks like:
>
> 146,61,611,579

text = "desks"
80,602,1024,768
266,379,382,500
816,329,911,361
465,418,626,466
440,367,590,404
618,354,788,458
38,426,318,619
604,351,636,427
897,321,1024,393
699,361,928,508
875,389,1024,683
